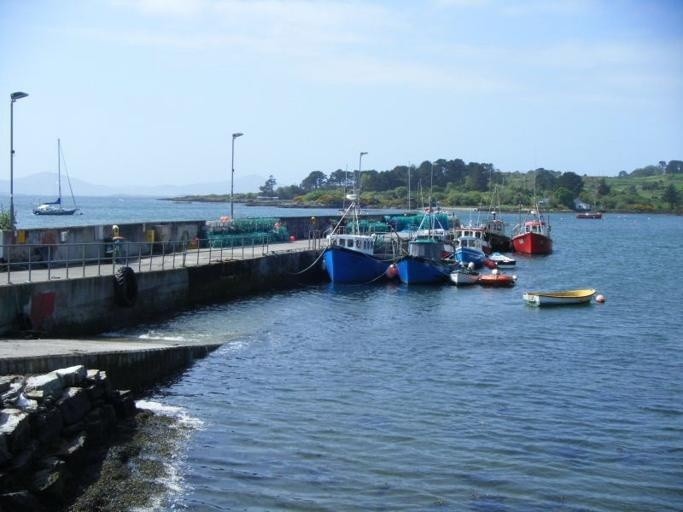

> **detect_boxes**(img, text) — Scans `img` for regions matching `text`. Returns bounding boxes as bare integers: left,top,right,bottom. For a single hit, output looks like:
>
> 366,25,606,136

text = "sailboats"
323,170,553,285
34,138,80,214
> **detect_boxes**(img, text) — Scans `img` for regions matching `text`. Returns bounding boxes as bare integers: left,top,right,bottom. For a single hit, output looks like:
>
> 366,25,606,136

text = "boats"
577,210,602,219
524,290,598,310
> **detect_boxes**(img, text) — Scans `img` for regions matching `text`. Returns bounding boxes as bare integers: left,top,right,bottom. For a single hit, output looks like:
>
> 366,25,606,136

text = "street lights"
10,92,30,230
230,131,245,216
357,151,369,209
